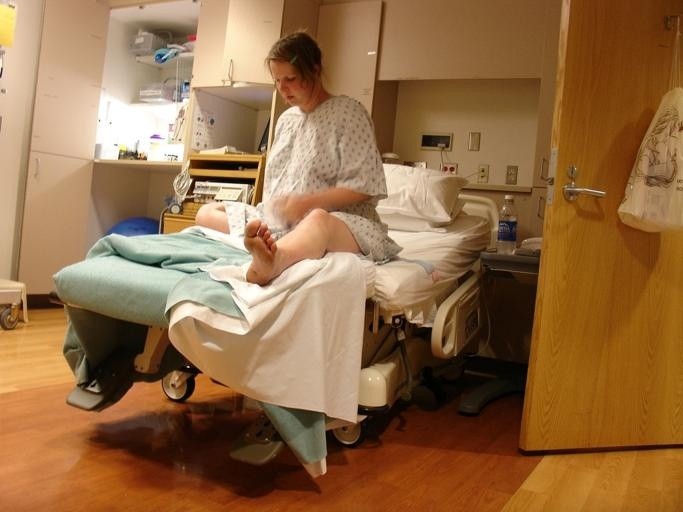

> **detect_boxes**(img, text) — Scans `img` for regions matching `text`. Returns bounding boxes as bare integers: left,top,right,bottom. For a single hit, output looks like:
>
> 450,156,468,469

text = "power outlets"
438,162,518,185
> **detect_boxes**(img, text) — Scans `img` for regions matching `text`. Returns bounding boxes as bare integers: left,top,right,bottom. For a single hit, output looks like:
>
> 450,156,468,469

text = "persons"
193,30,404,286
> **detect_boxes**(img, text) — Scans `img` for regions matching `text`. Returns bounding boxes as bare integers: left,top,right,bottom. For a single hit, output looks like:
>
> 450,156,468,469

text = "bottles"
497,193,517,256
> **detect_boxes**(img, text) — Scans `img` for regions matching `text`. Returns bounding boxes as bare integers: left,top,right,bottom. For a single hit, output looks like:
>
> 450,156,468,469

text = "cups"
147,135,163,162
381,152,399,164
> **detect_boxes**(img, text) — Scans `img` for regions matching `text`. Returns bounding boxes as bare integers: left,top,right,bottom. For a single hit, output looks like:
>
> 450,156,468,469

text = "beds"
47,189,501,473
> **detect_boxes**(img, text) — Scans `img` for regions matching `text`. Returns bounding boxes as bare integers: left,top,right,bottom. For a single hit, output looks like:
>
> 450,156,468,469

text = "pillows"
376,194,467,233
368,157,466,223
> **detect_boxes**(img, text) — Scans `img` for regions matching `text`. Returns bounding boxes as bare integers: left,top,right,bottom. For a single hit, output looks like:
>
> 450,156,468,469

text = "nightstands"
476,250,546,364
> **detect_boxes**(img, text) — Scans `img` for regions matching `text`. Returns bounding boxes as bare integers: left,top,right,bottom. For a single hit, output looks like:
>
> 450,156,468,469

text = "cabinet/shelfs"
190,0,318,112
311,0,399,163
16,0,258,295
156,152,266,236
